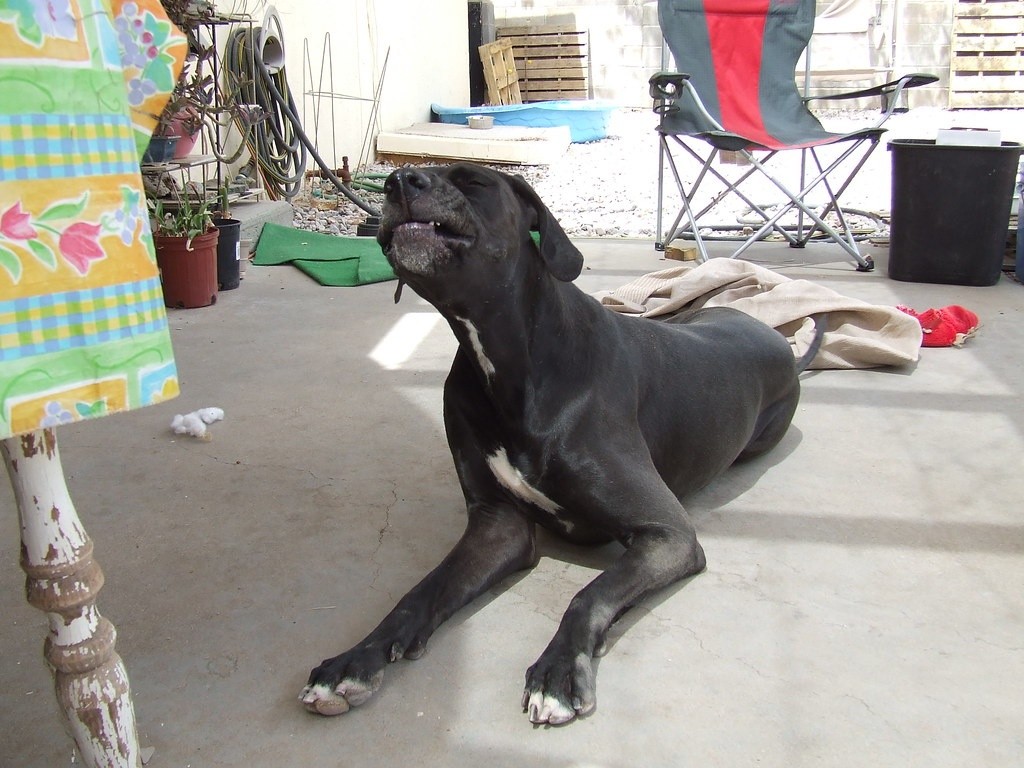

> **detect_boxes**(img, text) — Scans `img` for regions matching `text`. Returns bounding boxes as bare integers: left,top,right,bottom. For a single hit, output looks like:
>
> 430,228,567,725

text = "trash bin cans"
888,138,1024,287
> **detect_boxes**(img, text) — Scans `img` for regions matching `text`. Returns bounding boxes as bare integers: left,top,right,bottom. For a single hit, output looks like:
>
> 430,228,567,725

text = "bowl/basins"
465,115,495,129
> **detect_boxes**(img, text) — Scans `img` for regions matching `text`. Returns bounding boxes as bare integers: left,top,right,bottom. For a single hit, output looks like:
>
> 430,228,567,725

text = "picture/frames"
213,218,242,290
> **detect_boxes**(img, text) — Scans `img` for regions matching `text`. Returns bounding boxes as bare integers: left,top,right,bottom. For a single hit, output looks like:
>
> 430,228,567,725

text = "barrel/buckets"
210,217,242,291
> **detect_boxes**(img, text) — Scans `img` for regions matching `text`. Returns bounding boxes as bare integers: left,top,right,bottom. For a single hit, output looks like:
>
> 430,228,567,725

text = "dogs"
297,162,825,726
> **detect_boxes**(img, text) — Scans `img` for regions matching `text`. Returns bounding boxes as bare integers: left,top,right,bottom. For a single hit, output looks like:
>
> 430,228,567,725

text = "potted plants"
141,169,220,309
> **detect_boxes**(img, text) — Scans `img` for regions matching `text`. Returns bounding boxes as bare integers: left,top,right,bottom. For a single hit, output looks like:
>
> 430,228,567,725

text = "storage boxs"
142,135,182,162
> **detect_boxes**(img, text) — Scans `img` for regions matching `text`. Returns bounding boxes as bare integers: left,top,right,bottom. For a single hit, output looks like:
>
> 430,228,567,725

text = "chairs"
649,0,939,272
